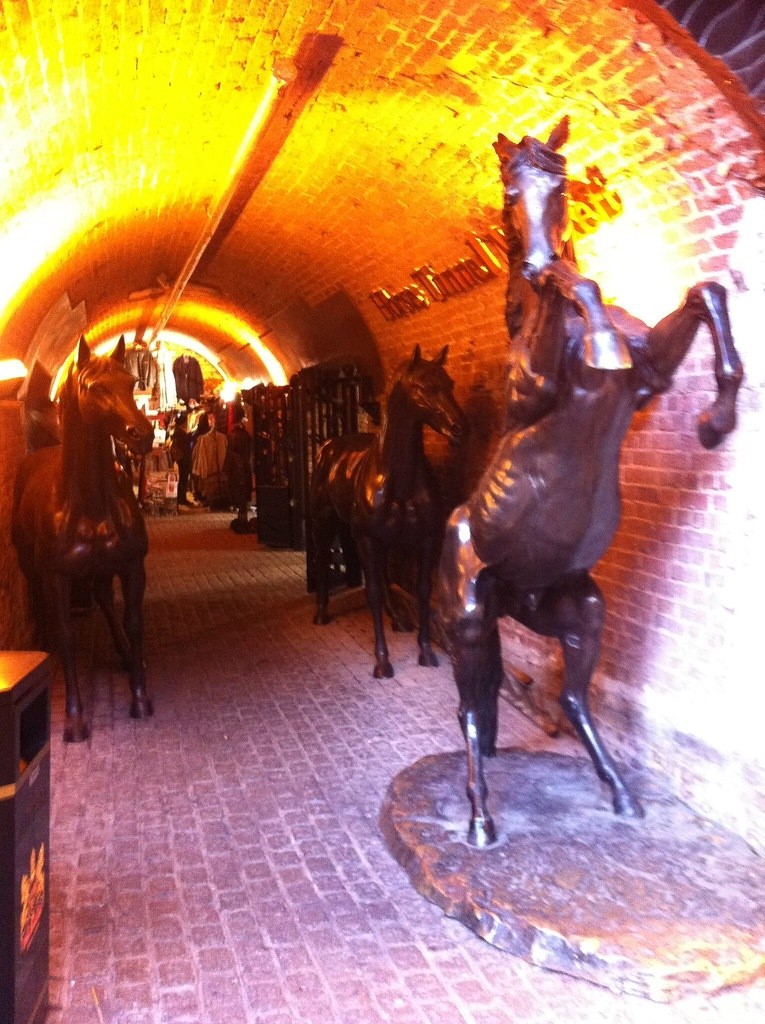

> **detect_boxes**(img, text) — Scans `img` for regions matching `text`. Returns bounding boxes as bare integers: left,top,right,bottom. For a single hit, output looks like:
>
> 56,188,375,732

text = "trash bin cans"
0,645,58,1024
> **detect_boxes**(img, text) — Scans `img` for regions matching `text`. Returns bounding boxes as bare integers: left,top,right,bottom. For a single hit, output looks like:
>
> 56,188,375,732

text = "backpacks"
170,429,185,462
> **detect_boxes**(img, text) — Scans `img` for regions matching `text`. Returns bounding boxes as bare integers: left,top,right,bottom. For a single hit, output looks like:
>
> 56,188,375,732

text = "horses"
431,115,747,849
7,328,162,749
309,339,469,680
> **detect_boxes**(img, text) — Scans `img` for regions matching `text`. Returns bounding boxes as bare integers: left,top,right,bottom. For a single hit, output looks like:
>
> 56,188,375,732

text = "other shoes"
178,500,193,506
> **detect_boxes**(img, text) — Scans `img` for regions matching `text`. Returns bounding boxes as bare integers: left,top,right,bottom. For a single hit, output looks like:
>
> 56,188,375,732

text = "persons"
169,416,202,508
222,406,253,506
